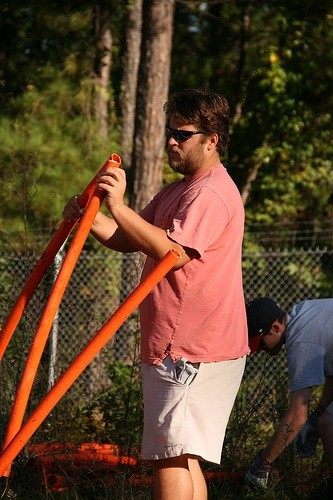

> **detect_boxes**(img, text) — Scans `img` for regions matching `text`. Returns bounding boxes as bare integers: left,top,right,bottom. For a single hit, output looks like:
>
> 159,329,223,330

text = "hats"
245,298,278,354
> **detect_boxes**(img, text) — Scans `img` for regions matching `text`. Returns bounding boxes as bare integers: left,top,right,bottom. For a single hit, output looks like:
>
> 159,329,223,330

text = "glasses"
165,125,206,142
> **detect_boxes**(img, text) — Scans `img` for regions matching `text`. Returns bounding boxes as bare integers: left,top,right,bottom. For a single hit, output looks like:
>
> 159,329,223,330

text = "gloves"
245,457,271,487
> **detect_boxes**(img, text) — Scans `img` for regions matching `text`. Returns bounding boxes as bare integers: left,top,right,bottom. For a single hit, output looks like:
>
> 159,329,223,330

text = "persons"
61,87,251,500
246,298,333,487
